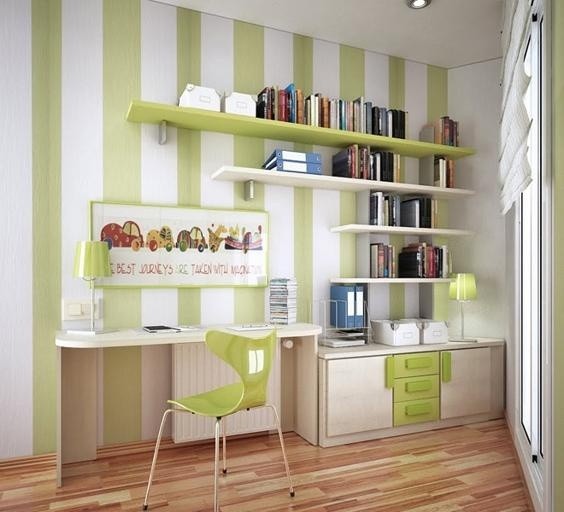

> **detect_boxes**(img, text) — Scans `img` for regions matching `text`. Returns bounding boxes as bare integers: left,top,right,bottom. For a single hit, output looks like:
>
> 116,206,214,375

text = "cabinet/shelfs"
125,98,477,284
318,338,505,448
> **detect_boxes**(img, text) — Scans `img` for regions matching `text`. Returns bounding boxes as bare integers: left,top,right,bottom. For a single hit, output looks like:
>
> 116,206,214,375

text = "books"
253,83,410,184
369,190,452,278
418,115,459,188
269,277,297,324
143,324,171,332
317,281,373,349
155,327,181,334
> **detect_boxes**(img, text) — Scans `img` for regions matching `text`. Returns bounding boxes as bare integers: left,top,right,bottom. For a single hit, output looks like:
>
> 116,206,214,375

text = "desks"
55,323,323,490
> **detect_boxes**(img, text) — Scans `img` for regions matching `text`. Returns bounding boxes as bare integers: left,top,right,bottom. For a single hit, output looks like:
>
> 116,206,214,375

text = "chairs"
142,328,294,512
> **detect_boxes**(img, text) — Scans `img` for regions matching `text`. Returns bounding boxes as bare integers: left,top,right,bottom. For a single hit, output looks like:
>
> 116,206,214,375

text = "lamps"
449,273,477,343
72,241,111,334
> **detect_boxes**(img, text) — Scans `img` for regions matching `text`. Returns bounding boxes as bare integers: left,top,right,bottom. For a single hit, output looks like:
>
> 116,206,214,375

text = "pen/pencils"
242,325,268,327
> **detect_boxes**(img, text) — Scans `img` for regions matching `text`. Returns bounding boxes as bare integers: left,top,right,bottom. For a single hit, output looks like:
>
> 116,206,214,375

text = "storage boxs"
371,319,420,347
178,84,221,112
401,317,448,345
220,91,258,117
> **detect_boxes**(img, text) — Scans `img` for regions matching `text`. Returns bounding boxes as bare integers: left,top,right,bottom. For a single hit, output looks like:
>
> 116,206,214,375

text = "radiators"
171,337,282,444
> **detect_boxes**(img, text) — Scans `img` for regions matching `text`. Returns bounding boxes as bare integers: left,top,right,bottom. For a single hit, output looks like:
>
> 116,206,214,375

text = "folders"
330,285,365,327
263,149,324,174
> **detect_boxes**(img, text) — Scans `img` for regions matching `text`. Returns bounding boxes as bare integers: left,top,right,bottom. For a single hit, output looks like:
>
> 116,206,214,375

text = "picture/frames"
90,201,270,289
